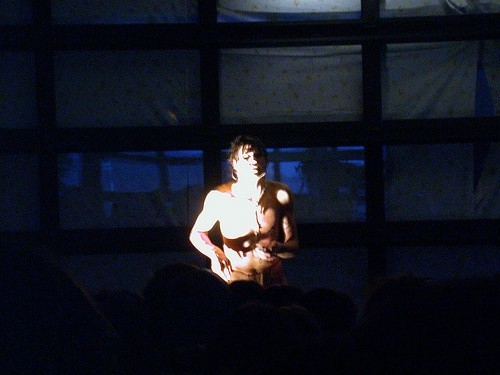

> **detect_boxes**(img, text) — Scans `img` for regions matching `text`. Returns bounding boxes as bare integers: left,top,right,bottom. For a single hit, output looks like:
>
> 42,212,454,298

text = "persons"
187,133,300,288
0,257,500,375
